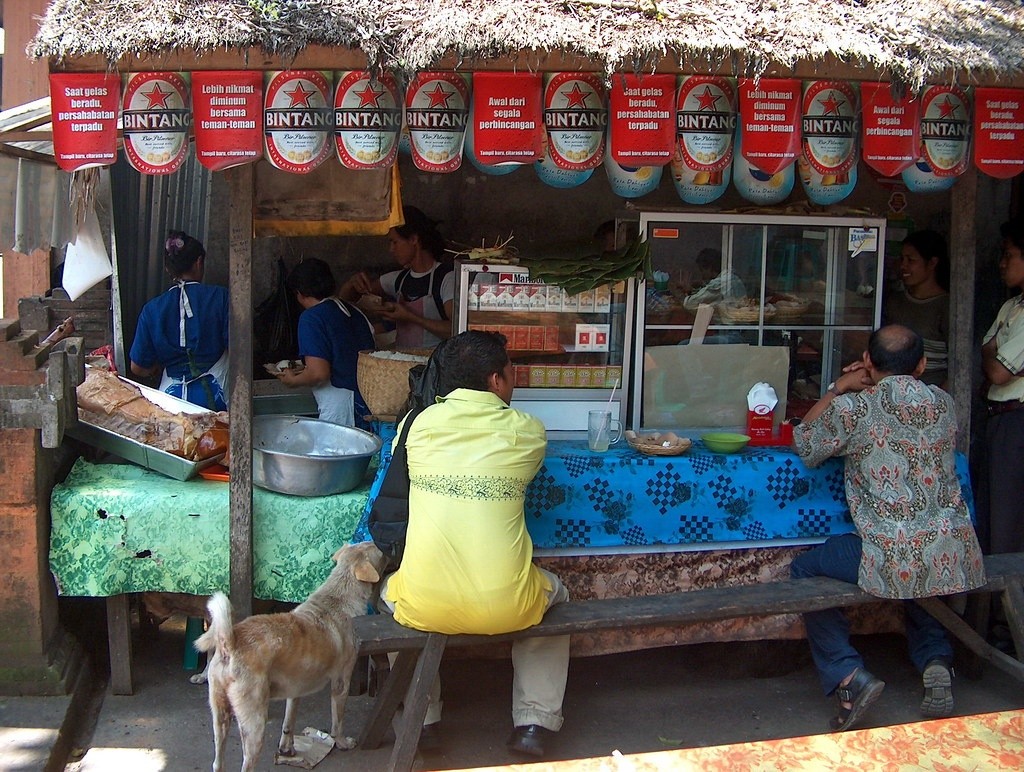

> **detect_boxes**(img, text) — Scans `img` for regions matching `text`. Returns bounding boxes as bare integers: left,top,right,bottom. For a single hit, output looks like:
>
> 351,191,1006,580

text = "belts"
985,400,1024,417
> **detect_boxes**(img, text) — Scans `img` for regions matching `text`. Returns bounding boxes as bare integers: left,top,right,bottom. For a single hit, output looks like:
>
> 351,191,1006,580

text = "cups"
588,410,623,452
655,281,668,289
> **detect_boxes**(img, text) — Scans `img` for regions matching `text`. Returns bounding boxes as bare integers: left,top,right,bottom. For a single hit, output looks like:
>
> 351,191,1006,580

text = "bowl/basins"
702,433,752,453
232,414,383,496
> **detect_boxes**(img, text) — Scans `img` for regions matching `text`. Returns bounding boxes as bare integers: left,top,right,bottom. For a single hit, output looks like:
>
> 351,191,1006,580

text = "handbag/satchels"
368,404,427,565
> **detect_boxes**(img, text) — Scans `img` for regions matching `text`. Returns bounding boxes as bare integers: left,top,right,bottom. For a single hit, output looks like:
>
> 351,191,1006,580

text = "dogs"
191,541,391,772
141,590,279,686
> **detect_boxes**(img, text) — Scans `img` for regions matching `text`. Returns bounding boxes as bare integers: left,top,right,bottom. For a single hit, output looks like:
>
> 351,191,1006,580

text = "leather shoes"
419,722,442,750
505,724,552,757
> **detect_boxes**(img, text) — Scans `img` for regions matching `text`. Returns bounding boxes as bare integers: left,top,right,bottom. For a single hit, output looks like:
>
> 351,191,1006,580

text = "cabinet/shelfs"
632,211,887,440
453,259,637,433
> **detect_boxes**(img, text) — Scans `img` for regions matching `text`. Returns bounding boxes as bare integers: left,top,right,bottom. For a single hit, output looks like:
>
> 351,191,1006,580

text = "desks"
53,424,978,698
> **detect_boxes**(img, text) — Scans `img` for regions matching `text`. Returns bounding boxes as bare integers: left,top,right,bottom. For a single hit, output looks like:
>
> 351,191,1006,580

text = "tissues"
745,380,792,446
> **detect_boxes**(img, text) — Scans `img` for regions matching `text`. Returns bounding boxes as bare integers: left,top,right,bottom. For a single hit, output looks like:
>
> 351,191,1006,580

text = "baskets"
721,311,777,323
625,430,691,455
765,303,812,324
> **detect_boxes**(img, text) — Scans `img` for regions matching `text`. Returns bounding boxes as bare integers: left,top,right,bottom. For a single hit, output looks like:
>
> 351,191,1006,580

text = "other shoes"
983,617,1015,655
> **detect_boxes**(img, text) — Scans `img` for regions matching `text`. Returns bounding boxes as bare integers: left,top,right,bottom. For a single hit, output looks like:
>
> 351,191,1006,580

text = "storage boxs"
466,272,622,389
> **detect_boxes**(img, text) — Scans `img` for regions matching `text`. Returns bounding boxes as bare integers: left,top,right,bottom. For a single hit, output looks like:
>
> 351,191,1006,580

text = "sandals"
923,660,956,717
831,671,884,734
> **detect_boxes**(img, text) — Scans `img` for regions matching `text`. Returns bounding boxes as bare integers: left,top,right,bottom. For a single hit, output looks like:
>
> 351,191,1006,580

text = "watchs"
827,382,841,396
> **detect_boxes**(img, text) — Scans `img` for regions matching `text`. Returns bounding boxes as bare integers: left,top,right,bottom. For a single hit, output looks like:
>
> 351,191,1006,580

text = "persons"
679,248,747,345
338,205,455,350
380,329,570,756
278,258,376,432
130,228,228,412
881,230,949,392
981,219,1024,554
792,324,987,732
647,288,673,346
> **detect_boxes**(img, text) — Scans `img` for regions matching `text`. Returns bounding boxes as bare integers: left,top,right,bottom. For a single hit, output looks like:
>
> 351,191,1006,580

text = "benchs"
350,551,1024,772
